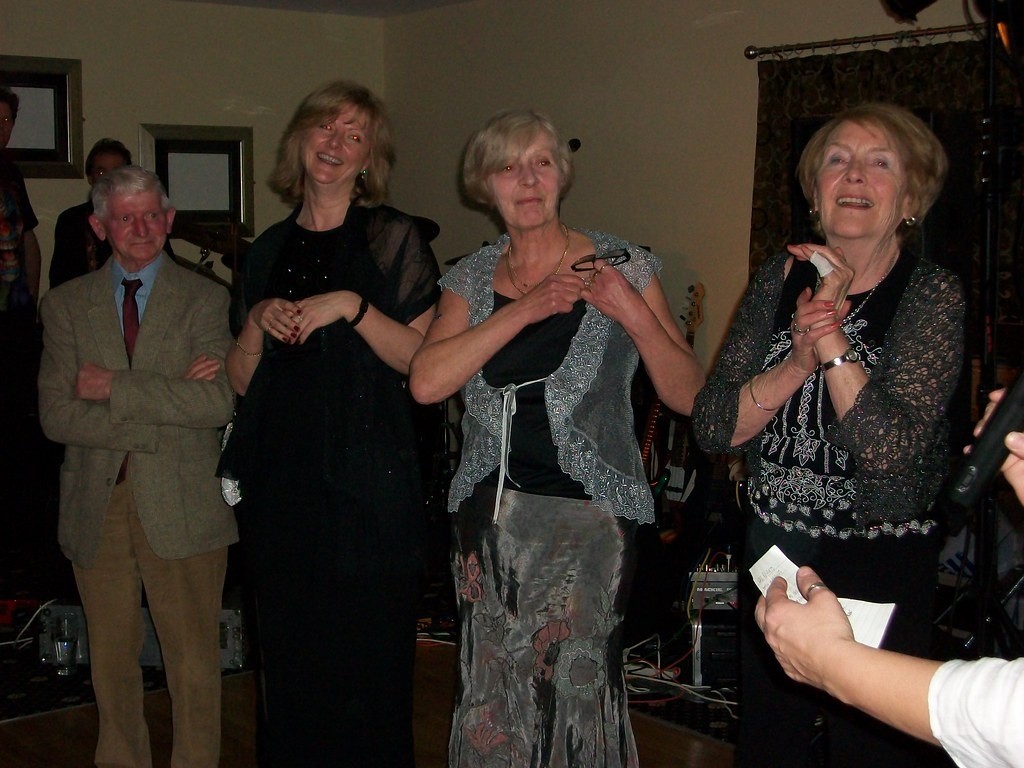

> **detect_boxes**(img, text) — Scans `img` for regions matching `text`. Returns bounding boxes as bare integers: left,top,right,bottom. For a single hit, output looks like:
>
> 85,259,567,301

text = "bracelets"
349,299,369,328
750,375,780,411
236,338,263,357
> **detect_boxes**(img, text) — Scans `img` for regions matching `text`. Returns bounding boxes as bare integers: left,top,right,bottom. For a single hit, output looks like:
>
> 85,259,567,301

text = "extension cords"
623,663,682,680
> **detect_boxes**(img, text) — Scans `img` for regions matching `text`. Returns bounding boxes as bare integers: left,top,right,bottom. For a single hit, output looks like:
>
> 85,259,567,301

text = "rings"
267,319,273,332
296,315,303,321
791,311,810,335
585,263,609,288
807,582,828,591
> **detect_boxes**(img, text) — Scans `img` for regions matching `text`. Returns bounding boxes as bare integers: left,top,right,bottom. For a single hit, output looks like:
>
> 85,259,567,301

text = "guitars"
648,279,710,516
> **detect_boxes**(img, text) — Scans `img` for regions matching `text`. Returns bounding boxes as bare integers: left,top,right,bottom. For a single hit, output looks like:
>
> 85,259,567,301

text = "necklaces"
817,248,898,326
506,221,570,296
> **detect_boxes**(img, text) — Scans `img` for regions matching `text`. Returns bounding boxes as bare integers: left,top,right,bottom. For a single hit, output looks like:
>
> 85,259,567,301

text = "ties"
113,278,143,485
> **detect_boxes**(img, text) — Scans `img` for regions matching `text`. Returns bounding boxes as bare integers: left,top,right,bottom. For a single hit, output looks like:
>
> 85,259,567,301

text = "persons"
225,80,441,768
410,107,704,768
755,390,1024,768
691,102,971,768
40,138,241,768
0,85,134,603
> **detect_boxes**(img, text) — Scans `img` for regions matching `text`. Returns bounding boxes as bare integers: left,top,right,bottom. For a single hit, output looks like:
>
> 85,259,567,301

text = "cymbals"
172,255,234,290
169,216,251,256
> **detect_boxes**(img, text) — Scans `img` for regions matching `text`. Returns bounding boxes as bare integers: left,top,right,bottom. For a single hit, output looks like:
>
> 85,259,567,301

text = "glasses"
2,116,13,123
93,169,105,177
571,248,631,272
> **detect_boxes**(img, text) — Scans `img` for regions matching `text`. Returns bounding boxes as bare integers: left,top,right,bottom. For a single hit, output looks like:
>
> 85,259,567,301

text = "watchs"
820,344,858,373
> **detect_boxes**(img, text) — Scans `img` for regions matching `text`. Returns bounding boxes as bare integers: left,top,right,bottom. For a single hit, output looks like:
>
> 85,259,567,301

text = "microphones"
940,364,1024,509
